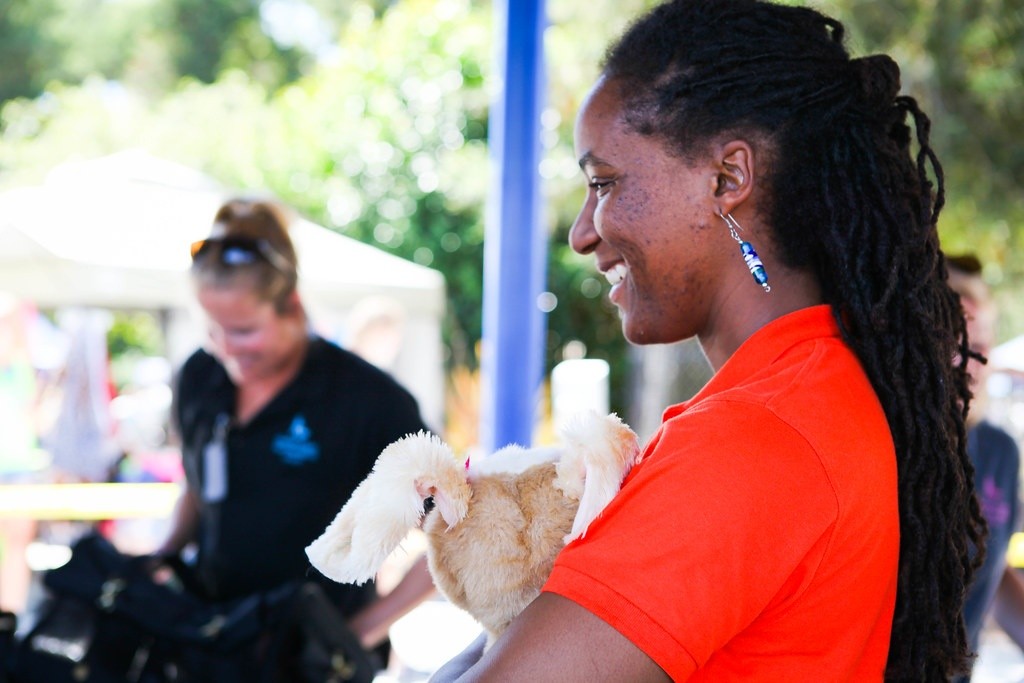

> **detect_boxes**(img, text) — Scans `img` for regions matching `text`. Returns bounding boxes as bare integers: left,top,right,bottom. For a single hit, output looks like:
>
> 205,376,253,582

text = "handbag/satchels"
12,530,374,683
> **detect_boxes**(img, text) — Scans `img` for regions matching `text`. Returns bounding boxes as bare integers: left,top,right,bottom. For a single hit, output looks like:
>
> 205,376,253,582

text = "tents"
0,82,450,602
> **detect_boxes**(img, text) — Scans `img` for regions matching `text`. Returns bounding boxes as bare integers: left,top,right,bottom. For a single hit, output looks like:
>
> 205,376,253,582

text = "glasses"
191,236,295,281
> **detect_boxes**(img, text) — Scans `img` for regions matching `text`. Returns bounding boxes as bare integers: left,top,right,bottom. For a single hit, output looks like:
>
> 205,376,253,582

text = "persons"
158,199,435,683
945,254,1019,683
431,0,990,682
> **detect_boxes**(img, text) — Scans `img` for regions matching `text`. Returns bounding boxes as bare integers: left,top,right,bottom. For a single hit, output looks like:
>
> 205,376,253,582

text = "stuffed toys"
304,412,640,659
